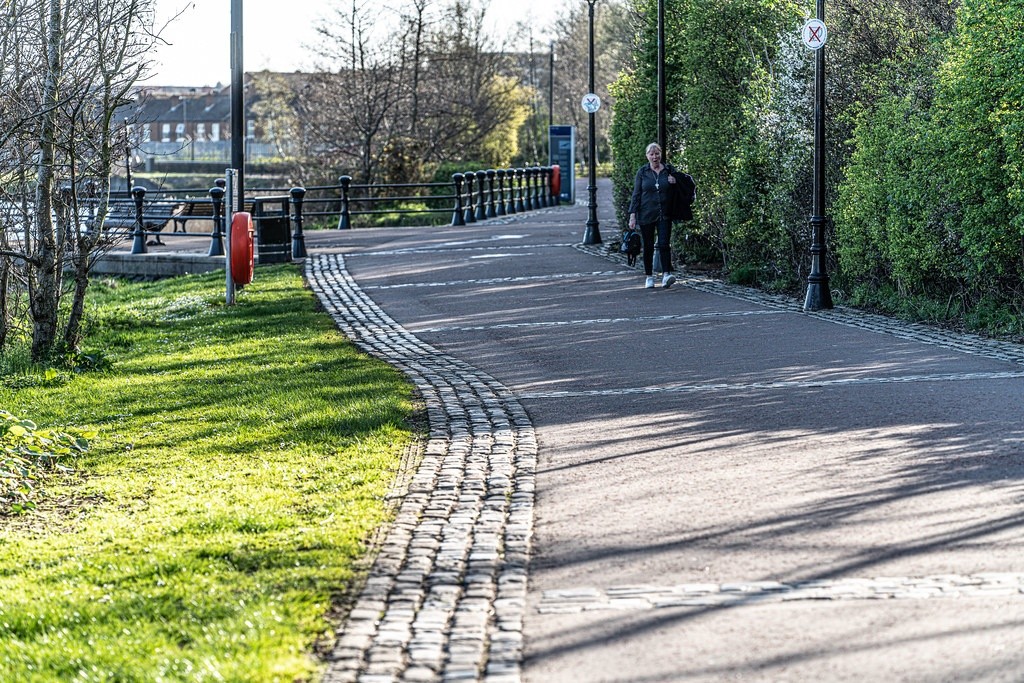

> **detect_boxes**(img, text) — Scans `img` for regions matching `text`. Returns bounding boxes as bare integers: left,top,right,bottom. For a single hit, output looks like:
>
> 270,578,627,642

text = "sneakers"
645,276,654,288
661,274,676,288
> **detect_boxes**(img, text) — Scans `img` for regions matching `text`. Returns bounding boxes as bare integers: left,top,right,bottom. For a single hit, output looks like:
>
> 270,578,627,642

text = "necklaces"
652,171,660,189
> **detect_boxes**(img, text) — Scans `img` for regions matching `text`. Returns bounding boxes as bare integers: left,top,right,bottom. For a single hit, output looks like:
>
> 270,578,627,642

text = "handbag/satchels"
622,226,641,267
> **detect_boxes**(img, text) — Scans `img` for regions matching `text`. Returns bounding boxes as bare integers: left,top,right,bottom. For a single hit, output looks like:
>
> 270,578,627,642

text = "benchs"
173,195,257,237
85,199,179,246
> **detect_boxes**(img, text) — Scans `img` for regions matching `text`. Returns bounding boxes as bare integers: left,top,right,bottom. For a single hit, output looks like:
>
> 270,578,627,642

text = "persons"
627,142,677,288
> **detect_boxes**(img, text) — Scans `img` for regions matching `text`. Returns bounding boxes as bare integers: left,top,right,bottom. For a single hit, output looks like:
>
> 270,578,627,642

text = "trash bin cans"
254,194,293,265
